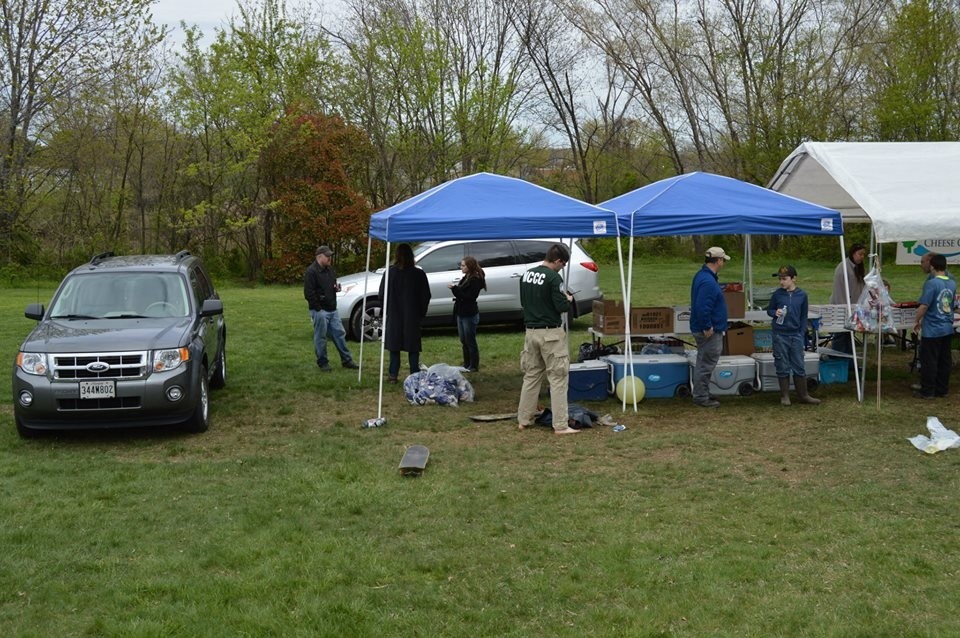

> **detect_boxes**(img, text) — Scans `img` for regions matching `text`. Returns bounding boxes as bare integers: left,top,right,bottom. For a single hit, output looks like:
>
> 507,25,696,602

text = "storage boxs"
724,291,745,319
820,358,849,384
722,320,756,356
568,361,609,402
592,298,631,335
673,306,692,334
809,305,917,325
631,308,674,334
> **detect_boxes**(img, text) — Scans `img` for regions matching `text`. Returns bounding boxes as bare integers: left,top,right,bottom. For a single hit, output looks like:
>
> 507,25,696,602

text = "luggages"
578,327,614,364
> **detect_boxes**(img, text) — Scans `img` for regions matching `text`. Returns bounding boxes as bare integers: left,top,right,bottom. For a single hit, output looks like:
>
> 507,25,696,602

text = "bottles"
776,305,787,325
847,285,897,334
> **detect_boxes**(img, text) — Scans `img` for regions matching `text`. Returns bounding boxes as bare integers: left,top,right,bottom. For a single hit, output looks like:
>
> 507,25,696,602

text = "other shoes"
387,375,398,385
693,398,719,407
912,391,949,400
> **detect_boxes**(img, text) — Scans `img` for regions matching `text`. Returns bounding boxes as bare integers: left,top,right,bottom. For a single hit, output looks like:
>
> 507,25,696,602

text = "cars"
334,238,604,342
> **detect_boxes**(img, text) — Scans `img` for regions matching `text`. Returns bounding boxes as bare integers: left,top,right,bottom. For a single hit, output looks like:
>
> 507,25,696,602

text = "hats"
772,265,797,276
706,246,731,261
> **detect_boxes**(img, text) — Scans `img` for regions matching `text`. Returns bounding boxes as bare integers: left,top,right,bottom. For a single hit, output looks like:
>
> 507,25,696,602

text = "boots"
792,375,820,405
779,375,791,406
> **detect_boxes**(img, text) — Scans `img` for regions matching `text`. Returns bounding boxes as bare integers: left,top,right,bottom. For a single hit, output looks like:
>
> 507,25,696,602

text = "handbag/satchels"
844,265,898,333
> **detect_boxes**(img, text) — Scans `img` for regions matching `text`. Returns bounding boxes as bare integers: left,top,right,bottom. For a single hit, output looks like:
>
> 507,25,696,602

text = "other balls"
616,375,646,405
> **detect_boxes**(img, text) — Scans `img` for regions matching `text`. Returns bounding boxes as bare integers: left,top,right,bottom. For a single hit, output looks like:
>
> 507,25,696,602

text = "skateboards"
398,445,430,477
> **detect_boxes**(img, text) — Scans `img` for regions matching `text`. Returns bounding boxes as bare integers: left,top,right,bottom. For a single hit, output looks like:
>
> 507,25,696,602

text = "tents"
743,141,960,411
561,171,862,415
358,172,638,420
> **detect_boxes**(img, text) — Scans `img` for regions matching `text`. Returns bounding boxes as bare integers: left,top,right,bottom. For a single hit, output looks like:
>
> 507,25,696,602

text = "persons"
690,246,731,408
767,265,820,406
304,246,360,369
378,244,431,384
519,245,581,434
911,252,953,390
448,255,486,372
912,254,956,398
830,244,866,370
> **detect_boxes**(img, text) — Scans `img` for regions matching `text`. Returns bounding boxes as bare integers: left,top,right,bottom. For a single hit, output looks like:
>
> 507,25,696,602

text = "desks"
588,321,960,372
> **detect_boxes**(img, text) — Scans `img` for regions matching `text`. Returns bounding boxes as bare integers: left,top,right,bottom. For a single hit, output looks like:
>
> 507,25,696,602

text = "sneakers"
342,359,359,369
319,358,332,371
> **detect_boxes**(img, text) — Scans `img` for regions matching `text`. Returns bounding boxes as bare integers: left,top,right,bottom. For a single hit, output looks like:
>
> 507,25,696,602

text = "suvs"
11,249,227,436
